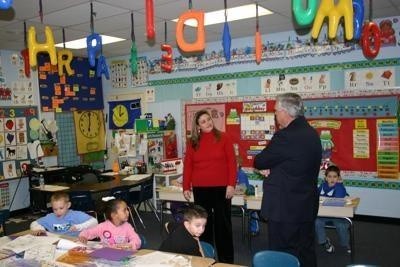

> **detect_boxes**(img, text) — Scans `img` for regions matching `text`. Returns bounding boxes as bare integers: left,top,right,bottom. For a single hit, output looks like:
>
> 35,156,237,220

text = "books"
0,232,138,266
322,197,347,207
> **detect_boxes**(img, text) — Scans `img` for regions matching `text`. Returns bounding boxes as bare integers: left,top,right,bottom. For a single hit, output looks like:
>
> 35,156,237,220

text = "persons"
169,174,192,224
252,91,323,267
315,164,351,256
28,191,99,238
156,203,210,258
182,108,239,264
236,161,260,237
77,198,142,250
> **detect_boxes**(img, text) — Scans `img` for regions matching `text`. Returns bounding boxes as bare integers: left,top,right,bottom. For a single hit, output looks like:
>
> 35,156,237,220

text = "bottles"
39,174,44,189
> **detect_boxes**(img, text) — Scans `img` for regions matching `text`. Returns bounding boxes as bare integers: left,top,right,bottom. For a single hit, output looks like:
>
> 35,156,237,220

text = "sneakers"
324,237,335,254
346,245,351,254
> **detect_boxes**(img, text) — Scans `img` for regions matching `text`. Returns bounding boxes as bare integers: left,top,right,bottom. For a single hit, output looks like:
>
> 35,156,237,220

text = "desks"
27,164,68,215
31,174,152,214
160,186,360,264
0,229,252,267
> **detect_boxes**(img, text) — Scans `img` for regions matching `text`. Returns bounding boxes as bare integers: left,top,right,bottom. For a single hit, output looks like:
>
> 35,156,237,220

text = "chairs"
253,251,300,267
55,165,161,229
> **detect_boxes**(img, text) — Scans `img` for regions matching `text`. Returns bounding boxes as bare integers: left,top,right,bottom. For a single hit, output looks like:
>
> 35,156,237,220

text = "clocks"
74,109,106,155
112,105,129,127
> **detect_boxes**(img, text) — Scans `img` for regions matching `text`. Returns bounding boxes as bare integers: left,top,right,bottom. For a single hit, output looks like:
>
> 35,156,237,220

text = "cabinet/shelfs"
154,174,177,214
